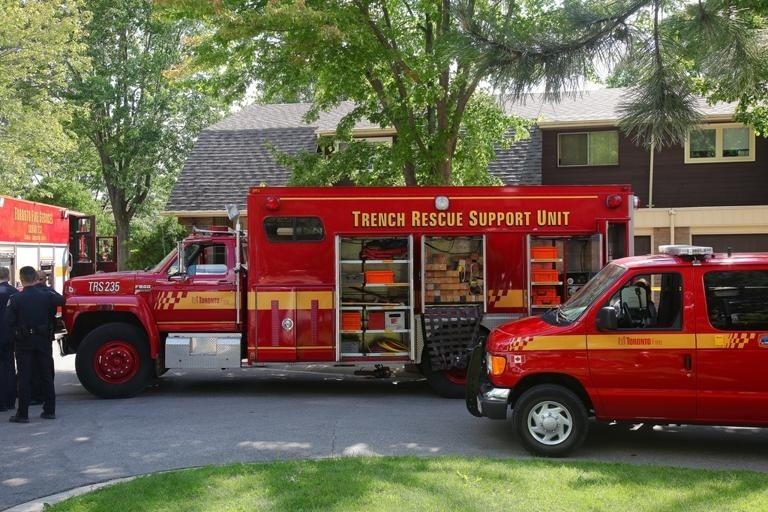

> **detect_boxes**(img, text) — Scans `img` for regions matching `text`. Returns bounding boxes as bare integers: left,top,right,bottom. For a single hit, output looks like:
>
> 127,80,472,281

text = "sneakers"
9,414,29,423
41,413,55,419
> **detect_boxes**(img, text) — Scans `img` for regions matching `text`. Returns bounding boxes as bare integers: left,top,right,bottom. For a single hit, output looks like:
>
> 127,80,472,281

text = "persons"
102,240,112,261
0,265,67,424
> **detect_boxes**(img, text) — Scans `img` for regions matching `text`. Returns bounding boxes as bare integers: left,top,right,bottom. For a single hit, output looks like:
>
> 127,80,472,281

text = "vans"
477,245,768,457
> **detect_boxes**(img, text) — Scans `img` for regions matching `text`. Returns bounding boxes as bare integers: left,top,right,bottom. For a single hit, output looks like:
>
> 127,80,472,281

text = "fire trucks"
58,183,640,399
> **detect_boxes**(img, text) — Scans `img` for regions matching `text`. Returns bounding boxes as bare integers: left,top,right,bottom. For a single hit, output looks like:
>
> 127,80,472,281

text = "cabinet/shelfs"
334,234,415,363
526,234,569,317
